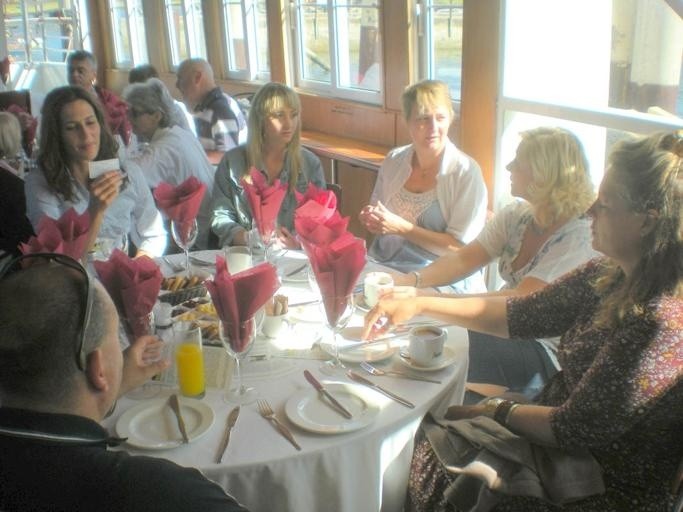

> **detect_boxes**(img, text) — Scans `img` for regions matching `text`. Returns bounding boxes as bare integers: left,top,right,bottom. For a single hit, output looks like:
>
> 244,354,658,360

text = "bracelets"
412,272,420,288
485,398,519,429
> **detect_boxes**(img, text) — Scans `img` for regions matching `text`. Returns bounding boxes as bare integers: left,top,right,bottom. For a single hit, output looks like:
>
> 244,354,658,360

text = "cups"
167,318,207,399
408,322,448,368
82,229,397,375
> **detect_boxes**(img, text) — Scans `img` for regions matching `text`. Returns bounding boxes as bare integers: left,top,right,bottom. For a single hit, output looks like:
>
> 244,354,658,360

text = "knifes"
166,395,190,444
213,405,240,462
346,369,414,409
302,369,351,418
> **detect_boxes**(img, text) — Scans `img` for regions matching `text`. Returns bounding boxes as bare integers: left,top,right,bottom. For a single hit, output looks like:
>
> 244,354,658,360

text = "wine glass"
214,316,259,407
170,216,198,276
114,311,163,398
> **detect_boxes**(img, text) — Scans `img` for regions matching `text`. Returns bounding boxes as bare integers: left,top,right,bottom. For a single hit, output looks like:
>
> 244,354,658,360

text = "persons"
377,125,604,405
23,85,167,261
128,63,193,131
358,80,487,295
123,78,214,250
175,57,247,151
1,111,37,172
0,253,250,512
362,127,683,512
209,82,325,250
7,51,133,147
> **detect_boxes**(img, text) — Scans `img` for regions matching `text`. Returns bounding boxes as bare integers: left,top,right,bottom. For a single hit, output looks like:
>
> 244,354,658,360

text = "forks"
358,360,444,385
254,397,303,452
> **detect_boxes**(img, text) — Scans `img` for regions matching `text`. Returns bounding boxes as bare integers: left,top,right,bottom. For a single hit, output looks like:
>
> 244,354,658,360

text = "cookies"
189,322,219,341
160,272,204,293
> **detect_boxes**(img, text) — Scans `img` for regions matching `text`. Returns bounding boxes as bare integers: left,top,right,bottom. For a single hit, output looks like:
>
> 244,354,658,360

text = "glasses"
125,108,149,118
0,253,93,371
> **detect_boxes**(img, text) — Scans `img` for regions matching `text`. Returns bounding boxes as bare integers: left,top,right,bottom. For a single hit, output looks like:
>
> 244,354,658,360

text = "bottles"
111,131,149,160
1,137,39,173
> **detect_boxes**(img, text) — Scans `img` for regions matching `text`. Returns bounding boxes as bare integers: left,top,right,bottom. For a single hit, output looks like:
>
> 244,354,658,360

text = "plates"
286,382,385,435
398,345,455,372
114,394,214,448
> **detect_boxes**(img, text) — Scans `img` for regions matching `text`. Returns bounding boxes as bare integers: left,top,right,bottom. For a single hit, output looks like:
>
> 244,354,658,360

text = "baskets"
157,276,207,306
